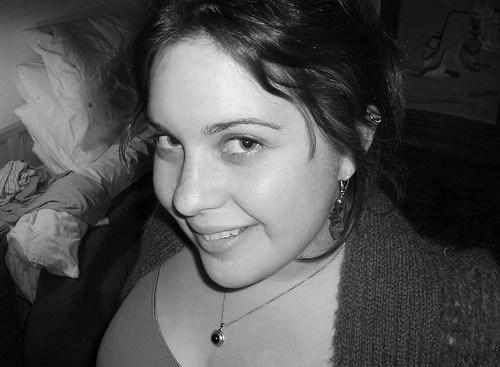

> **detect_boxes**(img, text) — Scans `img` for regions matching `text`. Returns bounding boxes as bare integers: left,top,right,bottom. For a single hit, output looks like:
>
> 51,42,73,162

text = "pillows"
12,104,79,175
15,60,111,170
23,3,150,165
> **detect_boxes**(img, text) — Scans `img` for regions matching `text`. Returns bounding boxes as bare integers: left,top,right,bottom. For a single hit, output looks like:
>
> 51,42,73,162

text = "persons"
95,0,500,365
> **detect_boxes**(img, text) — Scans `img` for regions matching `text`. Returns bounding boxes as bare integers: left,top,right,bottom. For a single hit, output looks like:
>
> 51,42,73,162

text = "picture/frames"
374,0,500,168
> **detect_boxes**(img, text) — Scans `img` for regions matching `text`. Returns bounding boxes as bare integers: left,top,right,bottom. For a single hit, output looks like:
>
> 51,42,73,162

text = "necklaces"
211,242,345,347
